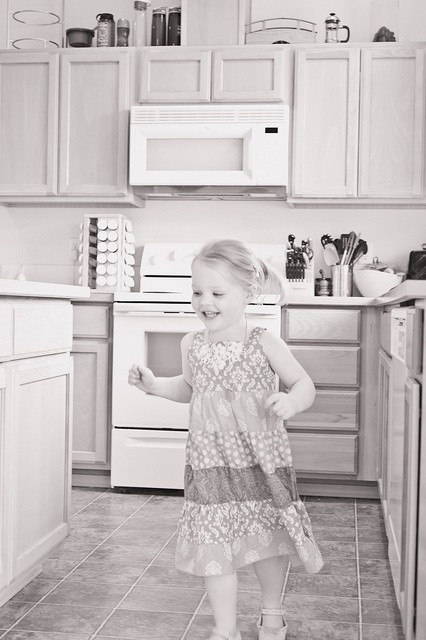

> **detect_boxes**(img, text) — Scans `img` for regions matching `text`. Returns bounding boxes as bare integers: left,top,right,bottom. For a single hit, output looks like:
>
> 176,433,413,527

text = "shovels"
340,234,350,254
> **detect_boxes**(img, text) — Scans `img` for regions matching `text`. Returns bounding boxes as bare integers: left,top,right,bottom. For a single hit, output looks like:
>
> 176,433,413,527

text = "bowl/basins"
354,270,403,298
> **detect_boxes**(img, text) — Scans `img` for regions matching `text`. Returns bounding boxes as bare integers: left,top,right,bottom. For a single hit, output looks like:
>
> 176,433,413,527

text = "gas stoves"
112,291,285,304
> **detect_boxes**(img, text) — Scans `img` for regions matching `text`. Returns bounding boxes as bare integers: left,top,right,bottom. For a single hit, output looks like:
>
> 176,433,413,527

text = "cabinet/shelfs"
0,45,144,208
289,40,425,207
137,44,290,104
65,298,109,490
0,296,75,606
279,307,386,501
376,303,421,640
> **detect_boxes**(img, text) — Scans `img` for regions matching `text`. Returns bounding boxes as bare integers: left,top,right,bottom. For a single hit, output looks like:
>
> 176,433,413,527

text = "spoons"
323,247,340,266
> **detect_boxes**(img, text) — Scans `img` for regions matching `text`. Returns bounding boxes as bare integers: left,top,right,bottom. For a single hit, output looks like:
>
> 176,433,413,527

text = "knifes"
286,252,306,282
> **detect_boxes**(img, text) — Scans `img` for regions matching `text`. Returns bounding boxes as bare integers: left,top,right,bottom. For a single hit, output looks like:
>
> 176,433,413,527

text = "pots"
66,28,94,47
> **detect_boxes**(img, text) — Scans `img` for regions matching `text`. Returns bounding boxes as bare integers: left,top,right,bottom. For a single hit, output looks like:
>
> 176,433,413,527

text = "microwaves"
129,104,289,201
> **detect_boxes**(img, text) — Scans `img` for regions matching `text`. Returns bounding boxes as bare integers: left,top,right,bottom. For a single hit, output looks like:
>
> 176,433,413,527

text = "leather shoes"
256,602,288,640
209,625,242,639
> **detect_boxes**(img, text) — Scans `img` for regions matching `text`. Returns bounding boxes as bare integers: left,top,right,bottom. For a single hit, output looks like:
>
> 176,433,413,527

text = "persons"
128,239,324,640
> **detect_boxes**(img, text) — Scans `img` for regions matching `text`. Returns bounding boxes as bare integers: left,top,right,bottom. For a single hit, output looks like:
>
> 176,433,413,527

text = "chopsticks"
339,233,361,264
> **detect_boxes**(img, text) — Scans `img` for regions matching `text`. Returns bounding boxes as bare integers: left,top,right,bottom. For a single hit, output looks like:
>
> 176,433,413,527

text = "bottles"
95,14,115,47
150,6,166,46
167,7,181,46
131,1,147,46
324,13,350,44
116,18,130,46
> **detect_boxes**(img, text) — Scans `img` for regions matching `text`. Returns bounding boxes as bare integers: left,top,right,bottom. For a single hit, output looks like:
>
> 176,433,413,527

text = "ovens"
111,303,280,490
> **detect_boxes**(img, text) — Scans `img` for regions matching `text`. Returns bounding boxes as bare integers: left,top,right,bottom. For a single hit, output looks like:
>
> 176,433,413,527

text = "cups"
331,265,353,297
